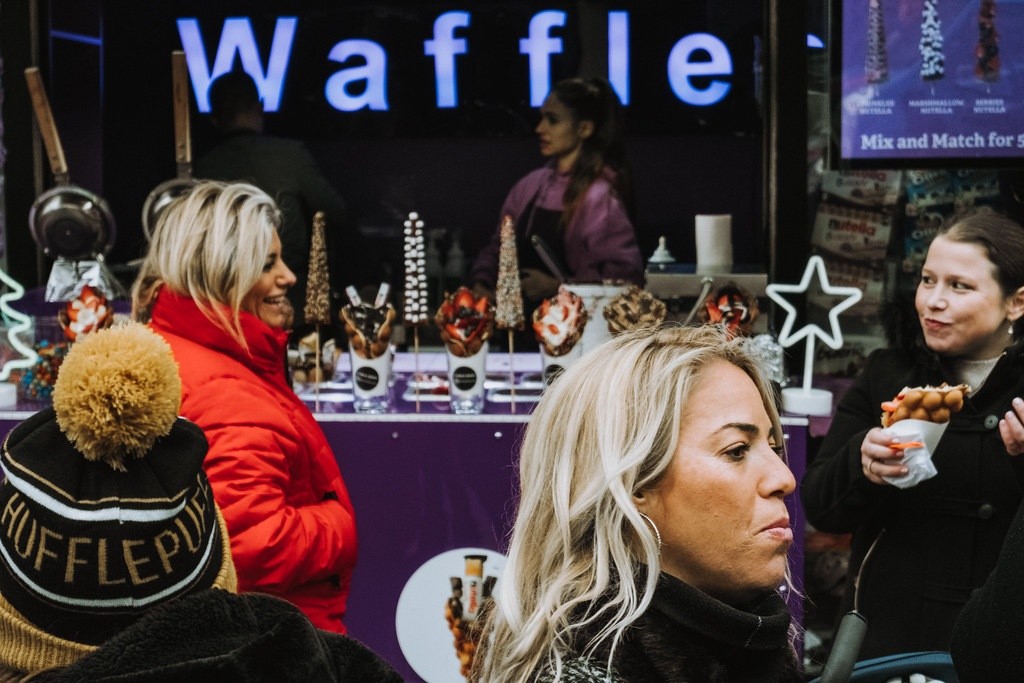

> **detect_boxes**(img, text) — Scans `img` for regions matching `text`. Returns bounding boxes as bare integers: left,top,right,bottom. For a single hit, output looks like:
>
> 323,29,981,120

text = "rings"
867,459,876,471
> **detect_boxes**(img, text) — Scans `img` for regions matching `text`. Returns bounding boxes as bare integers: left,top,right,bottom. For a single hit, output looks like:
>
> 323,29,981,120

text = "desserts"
602,289,668,337
57,285,115,341
878,383,972,424
531,290,588,355
339,283,396,359
697,282,760,340
434,286,497,356
291,332,341,384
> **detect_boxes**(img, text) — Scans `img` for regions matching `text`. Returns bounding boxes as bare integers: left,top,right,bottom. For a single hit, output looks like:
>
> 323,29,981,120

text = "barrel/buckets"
559,284,635,352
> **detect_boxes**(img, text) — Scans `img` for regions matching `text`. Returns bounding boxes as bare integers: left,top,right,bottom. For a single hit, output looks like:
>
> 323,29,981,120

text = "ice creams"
445,554,496,683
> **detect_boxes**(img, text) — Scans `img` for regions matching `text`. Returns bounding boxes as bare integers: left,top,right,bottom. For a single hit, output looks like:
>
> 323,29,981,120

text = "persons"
131,180,358,634
474,73,641,353
0,322,404,683
799,209,1024,683
469,319,815,682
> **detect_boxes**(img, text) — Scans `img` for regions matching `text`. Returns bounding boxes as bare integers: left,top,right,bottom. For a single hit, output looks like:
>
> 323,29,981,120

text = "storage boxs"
883,418,950,459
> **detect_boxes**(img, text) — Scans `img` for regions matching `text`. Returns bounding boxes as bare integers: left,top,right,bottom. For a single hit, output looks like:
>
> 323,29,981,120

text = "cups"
348,340,392,415
695,215,733,275
541,343,583,390
445,342,488,415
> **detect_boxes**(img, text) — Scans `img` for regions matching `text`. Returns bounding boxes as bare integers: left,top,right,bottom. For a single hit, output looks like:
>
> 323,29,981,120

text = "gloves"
520,268,559,307
472,275,496,301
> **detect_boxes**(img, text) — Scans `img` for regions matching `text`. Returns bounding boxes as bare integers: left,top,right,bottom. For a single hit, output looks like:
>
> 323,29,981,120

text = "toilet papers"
694,214,732,268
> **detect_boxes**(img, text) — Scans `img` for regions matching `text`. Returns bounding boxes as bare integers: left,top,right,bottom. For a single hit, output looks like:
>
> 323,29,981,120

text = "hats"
0,322,238,671
